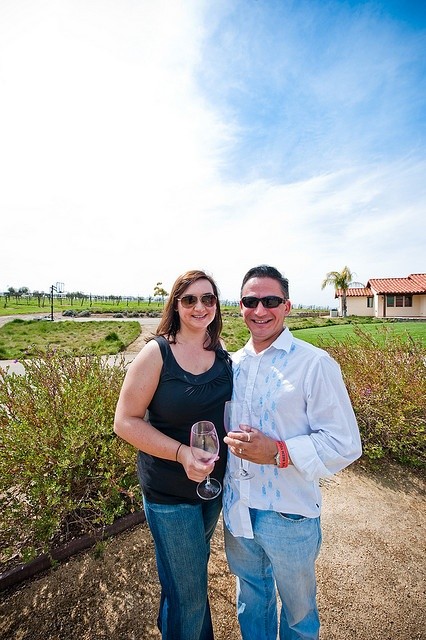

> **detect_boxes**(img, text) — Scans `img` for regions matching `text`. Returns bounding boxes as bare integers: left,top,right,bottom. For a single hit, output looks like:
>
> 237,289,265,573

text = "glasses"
175,295,218,307
241,296,285,308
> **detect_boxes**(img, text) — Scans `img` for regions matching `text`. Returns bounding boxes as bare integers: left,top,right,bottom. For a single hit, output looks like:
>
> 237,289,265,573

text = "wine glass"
223,400,255,481
190,420,222,502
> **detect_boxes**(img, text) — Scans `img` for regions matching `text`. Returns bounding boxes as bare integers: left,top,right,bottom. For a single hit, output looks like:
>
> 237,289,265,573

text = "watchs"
274,440,290,468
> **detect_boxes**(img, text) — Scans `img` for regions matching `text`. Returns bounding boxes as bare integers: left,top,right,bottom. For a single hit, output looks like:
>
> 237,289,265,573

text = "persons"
114,271,233,640
224,266,362,640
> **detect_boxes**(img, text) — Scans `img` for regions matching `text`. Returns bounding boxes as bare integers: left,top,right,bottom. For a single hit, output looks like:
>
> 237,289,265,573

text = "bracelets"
176,443,183,461
275,441,288,468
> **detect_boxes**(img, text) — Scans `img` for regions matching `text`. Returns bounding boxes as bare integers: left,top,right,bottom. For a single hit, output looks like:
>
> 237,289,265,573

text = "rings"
239,448,242,453
247,433,250,441
